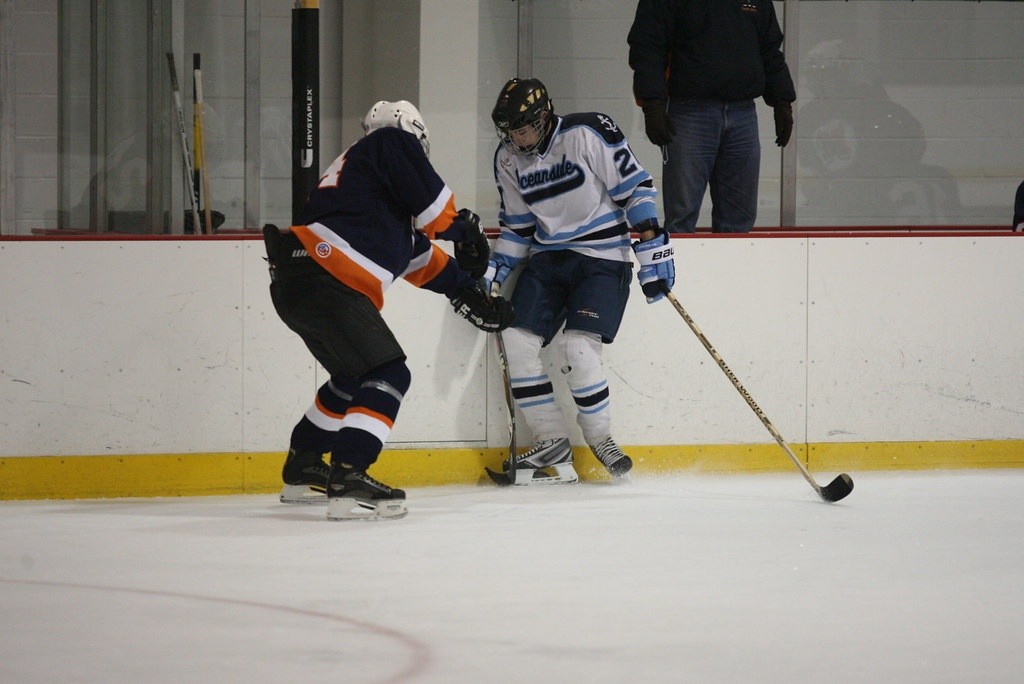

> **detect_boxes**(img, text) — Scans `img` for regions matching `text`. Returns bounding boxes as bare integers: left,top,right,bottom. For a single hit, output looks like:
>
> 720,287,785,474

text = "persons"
263,100,512,523
482,77,676,486
793,40,927,224
79,98,227,211
627,0,796,232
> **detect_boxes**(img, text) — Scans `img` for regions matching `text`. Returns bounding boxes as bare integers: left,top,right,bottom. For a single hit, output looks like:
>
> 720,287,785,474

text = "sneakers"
503,437,579,486
326,460,408,522
588,434,633,482
280,448,330,506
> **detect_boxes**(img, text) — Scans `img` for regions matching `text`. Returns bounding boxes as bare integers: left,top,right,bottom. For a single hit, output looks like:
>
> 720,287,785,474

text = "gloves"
774,108,793,146
442,271,516,333
441,209,490,280
482,258,514,297
630,228,675,304
644,103,677,146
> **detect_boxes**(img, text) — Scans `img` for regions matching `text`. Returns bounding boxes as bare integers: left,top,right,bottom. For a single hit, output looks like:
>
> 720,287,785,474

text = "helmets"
491,77,554,156
364,99,429,159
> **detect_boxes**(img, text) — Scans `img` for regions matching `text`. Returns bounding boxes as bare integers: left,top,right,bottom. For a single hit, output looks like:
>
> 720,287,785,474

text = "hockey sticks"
166,53,213,235
476,278,517,486
661,285,855,504
